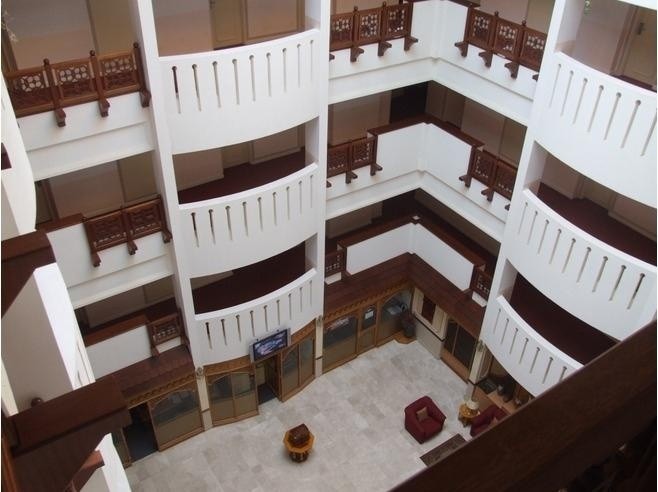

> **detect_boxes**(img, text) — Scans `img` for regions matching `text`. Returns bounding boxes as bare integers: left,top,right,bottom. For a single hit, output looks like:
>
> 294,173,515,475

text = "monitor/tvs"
249,328,291,364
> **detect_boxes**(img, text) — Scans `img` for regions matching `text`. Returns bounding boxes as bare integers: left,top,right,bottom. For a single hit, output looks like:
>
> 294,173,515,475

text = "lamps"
466,396,480,412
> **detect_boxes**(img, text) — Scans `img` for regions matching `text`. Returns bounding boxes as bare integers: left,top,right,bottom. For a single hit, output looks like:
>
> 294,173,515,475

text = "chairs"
401,393,447,445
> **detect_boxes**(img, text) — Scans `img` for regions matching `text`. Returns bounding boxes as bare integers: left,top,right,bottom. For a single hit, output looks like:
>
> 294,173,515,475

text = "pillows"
490,415,498,425
415,404,429,423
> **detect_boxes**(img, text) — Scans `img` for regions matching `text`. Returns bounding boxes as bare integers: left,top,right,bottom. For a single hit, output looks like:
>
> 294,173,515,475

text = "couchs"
470,404,503,439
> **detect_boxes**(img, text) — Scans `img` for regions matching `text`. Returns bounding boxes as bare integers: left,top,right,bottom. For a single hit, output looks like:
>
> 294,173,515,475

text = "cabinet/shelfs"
470,381,530,415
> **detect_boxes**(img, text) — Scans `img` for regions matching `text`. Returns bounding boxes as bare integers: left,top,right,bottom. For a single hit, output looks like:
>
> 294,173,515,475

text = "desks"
282,427,315,462
456,403,479,428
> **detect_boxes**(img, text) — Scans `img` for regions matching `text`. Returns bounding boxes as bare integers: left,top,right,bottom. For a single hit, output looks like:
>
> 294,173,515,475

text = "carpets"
418,432,469,467
